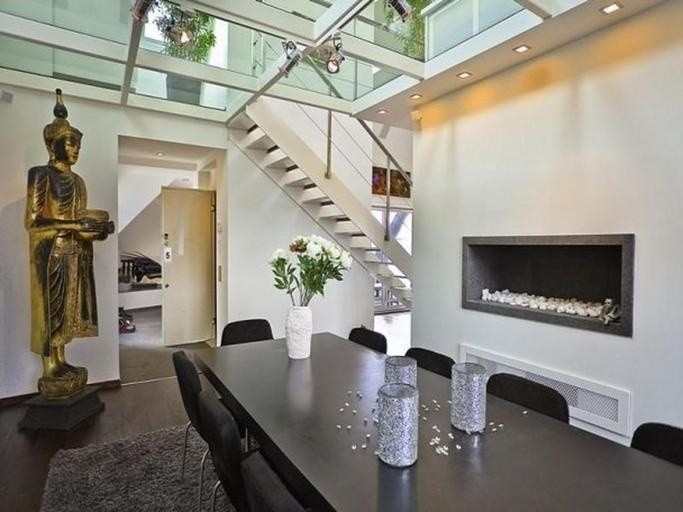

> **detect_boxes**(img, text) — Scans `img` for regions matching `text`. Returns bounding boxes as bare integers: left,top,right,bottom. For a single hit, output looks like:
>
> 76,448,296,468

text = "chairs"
185,392,310,511
404,346,454,380
347,324,388,354
219,317,274,346
485,372,568,425
172,349,250,511
631,420,682,467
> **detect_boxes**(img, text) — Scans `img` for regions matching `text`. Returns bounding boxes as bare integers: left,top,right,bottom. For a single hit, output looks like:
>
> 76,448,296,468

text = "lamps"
129,0,396,80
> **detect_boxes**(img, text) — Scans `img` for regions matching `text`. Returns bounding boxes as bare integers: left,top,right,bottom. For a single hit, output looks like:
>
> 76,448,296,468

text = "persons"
23,116,113,397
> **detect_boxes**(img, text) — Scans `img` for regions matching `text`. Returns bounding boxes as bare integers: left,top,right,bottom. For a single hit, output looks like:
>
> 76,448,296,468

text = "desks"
190,332,682,510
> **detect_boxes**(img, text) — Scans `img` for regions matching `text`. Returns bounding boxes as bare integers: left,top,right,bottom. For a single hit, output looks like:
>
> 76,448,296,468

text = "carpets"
38,423,261,512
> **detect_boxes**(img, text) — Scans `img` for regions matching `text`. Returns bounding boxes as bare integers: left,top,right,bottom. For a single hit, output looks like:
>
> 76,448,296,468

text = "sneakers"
119,307,135,332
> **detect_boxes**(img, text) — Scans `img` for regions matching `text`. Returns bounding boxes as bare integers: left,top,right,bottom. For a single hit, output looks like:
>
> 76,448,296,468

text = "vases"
285,306,314,359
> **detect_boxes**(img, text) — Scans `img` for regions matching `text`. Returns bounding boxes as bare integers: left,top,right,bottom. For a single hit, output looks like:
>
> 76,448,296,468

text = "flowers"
270,235,352,306
